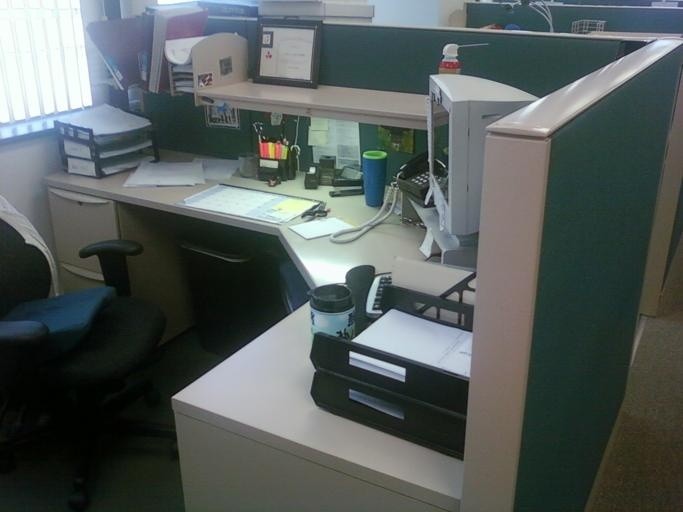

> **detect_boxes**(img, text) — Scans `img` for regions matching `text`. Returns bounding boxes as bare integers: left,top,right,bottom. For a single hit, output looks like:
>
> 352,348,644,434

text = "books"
148,1,374,94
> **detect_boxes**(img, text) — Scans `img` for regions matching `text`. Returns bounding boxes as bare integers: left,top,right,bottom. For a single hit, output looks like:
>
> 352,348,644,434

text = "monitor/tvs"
427,73,540,246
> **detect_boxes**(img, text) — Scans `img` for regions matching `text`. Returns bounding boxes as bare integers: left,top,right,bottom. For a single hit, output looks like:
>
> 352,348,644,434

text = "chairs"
0,196,180,512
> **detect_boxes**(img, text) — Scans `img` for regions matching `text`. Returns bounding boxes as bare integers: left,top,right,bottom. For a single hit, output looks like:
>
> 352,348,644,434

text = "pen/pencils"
260,133,289,159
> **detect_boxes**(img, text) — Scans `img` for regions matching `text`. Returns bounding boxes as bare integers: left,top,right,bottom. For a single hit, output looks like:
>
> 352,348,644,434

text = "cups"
361,150,387,206
309,284,356,344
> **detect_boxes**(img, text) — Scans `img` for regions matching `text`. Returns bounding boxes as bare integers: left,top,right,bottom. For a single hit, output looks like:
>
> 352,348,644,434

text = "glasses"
301,210,328,222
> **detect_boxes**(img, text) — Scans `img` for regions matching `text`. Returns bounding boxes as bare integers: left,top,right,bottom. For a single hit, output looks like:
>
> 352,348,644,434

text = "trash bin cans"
175,228,269,344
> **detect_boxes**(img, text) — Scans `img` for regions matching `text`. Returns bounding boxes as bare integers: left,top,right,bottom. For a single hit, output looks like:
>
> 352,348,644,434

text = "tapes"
306,167,315,175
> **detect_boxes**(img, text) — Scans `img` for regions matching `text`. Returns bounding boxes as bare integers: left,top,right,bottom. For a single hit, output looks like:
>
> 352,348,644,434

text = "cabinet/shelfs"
42,150,478,512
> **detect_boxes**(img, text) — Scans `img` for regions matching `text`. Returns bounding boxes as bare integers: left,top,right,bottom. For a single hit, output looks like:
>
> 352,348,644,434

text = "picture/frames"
253,22,322,89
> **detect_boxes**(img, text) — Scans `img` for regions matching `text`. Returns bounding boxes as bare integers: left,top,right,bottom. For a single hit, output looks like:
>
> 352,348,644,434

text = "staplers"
328,178,363,197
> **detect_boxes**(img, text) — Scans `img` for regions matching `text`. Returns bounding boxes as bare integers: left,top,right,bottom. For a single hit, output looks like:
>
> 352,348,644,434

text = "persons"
217,103,234,123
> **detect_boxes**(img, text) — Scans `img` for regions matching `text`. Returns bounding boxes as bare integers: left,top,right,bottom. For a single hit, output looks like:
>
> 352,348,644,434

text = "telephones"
392,154,449,197
